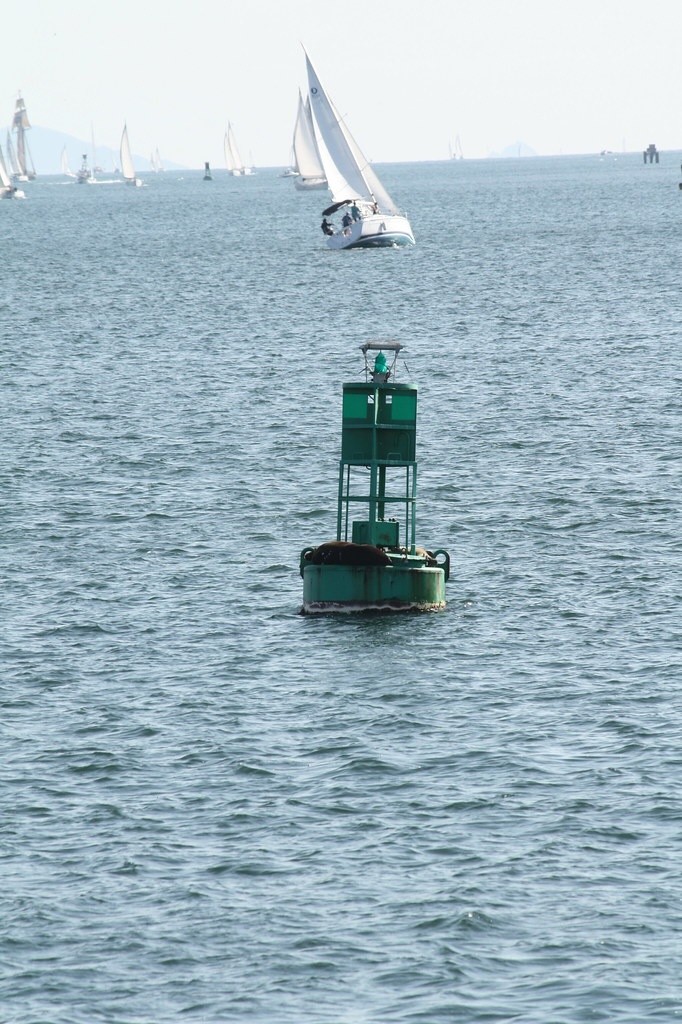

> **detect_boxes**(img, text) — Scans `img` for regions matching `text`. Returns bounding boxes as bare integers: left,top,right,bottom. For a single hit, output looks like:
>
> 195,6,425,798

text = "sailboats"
119,121,143,187
149,146,167,173
4,126,29,182
277,39,417,249
13,90,39,181
221,121,252,178
62,114,97,183
0,145,26,200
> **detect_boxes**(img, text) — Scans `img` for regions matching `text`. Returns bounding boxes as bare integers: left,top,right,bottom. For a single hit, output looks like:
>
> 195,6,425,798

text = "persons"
372,202,379,214
342,212,352,235
321,219,334,235
351,203,361,222
